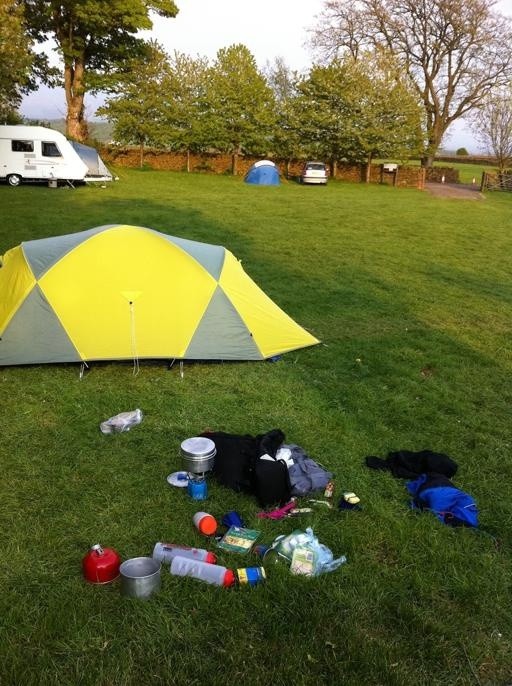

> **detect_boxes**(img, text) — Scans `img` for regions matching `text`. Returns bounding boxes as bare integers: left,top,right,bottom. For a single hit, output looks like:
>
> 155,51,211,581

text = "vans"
0,124,113,185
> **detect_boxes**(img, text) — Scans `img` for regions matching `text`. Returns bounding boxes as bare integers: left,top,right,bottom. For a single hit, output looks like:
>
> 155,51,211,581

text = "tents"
240,160,290,185
68,139,113,190
0,224,322,377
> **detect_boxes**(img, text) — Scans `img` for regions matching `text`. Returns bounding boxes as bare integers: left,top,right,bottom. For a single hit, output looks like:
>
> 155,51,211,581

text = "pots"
181,437,217,473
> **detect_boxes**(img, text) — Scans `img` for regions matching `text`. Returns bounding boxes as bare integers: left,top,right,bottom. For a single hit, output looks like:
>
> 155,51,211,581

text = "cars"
302,161,329,184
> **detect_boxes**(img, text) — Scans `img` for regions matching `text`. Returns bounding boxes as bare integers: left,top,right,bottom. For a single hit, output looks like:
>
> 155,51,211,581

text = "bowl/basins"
119,556,162,599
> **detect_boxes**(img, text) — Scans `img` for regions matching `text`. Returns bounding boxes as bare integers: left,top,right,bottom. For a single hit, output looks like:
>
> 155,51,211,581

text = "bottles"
193,511,217,534
153,542,216,564
170,555,234,586
